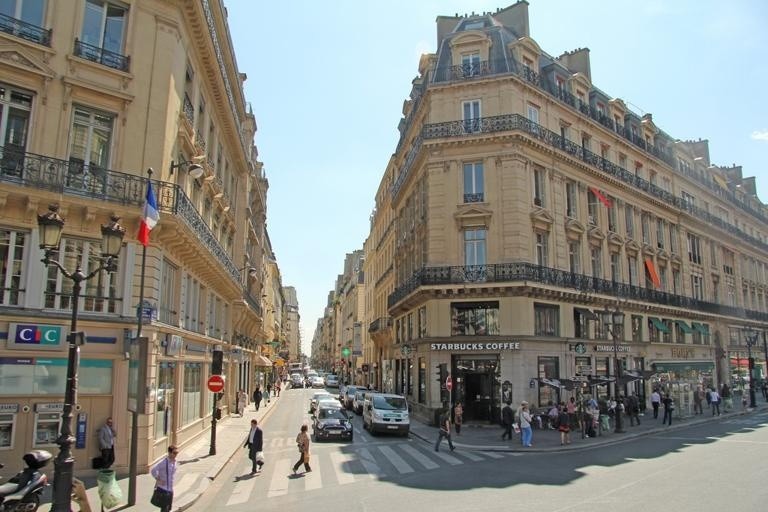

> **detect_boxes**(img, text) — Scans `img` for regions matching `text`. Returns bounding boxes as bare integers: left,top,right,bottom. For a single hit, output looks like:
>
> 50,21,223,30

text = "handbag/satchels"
304,450,310,465
255,450,265,463
511,422,521,434
149,485,174,511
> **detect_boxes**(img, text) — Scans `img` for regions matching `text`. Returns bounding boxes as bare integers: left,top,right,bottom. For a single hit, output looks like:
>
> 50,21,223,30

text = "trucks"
288,363,304,373
361,392,411,439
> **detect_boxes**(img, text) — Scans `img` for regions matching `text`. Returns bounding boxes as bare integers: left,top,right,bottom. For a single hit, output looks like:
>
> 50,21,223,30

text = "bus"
303,366,311,375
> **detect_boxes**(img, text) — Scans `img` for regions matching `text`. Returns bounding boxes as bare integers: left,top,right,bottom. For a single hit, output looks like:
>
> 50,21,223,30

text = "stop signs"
446,376,453,392
207,375,224,395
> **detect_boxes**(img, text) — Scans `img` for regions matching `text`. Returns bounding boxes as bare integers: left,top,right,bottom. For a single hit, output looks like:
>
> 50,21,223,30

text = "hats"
520,400,529,405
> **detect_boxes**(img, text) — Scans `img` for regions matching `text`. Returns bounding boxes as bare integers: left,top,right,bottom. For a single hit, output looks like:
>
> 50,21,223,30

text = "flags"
137,181,159,247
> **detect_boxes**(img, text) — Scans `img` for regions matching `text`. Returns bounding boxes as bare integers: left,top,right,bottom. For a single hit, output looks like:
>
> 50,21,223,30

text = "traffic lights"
211,348,224,375
344,346,349,357
434,361,448,385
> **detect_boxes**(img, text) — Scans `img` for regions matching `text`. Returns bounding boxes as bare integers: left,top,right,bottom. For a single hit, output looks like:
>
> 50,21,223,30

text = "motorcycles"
0,447,54,512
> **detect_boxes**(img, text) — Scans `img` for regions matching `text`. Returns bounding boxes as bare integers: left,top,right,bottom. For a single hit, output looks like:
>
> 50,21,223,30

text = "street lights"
345,324,356,384
742,323,758,409
601,300,627,433
36,199,127,512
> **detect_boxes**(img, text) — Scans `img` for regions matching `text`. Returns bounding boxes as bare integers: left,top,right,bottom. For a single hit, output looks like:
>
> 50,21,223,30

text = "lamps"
530,379,536,388
170,160,203,178
238,265,257,277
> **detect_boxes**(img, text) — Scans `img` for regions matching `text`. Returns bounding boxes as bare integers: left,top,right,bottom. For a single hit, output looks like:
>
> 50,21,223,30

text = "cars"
290,370,340,388
308,383,372,441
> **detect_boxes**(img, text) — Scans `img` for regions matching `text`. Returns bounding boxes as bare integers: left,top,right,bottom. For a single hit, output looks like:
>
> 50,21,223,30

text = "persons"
243,420,264,475
501,401,514,440
293,424,311,473
238,389,247,418
302,375,310,388
100,419,117,470
434,408,455,451
151,444,178,512
453,403,462,433
254,375,286,410
517,377,768,449
